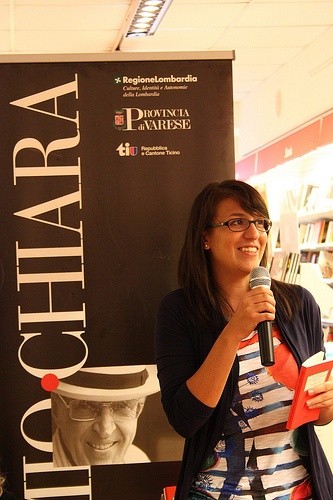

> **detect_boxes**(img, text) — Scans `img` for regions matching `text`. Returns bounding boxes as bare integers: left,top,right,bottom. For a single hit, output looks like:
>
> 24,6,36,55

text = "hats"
48,366,161,401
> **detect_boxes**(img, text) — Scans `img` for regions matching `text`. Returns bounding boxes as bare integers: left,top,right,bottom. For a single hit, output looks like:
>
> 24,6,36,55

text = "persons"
152,177,333,500
52,366,161,465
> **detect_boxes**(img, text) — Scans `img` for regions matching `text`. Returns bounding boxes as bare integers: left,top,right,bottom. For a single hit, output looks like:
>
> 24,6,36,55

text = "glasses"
58,396,143,422
206,217,272,233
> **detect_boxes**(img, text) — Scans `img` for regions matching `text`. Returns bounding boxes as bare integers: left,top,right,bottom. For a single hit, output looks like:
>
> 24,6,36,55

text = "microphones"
248,266,276,367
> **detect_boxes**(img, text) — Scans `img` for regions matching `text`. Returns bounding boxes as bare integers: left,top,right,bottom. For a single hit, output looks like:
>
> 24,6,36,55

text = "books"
284,350,333,431
269,217,333,344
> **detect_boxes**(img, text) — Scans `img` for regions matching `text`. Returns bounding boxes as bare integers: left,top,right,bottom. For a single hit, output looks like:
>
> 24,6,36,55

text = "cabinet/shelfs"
262,211,333,342
